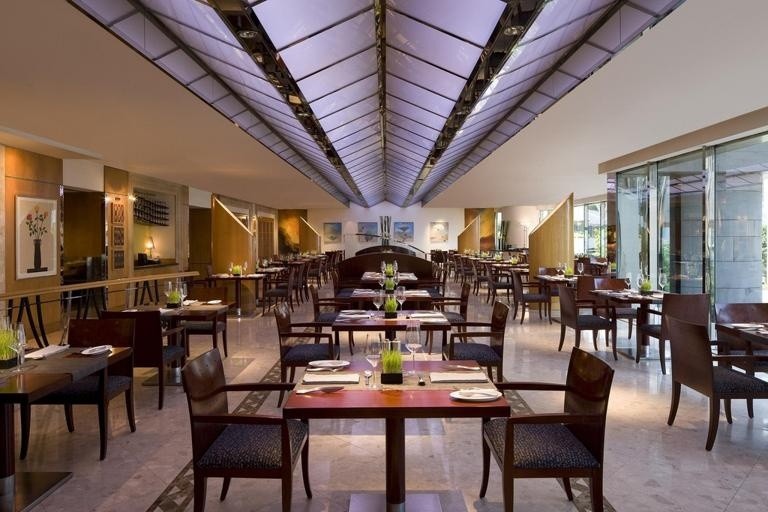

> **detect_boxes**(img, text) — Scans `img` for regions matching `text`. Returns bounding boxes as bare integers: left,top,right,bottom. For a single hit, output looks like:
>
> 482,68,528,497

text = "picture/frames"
429,221,449,243
112,248,126,271
12,194,61,283
393,221,414,244
358,221,378,243
109,202,125,225
112,224,127,248
321,222,342,244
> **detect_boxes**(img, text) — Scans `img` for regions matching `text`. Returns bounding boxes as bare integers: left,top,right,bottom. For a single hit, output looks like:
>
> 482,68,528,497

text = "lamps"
144,235,156,258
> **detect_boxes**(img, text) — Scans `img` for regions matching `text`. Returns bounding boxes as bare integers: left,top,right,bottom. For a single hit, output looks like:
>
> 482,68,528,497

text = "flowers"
24,204,49,240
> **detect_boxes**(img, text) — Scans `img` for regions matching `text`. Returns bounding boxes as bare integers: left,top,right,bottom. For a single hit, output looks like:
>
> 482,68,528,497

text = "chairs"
59,315,137,462
591,276,633,351
635,292,711,375
255,249,355,316
479,346,615,511
416,246,617,324
307,282,355,356
270,300,340,410
716,301,768,427
441,300,512,399
556,284,620,362
424,282,471,354
181,285,234,361
101,309,186,410
661,314,768,451
509,270,554,326
181,348,315,512
206,263,218,288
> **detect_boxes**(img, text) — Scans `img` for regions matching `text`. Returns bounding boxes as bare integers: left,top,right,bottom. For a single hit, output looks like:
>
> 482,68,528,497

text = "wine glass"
0,316,27,374
624,271,667,293
403,320,423,373
164,280,188,310
227,260,247,277
261,256,273,271
365,332,386,390
374,259,406,321
554,261,584,276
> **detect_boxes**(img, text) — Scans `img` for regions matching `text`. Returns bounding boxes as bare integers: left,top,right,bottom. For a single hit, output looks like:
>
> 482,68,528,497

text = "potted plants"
378,343,405,385
232,264,243,275
383,279,396,293
385,263,395,279
639,279,654,295
163,289,183,309
380,298,400,319
0,315,19,369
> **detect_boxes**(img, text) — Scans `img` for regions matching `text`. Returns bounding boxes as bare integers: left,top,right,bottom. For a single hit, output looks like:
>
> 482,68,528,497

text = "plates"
309,359,350,367
343,309,365,313
206,299,222,304
731,323,764,329
81,344,113,356
448,389,502,402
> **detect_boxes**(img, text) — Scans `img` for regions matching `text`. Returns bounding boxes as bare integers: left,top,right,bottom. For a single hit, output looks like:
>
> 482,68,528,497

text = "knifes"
418,372,425,386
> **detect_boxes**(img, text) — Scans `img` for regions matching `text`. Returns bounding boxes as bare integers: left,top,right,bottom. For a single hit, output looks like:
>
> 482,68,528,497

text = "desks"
330,307,451,354
196,273,266,318
277,357,511,511
713,321,767,349
102,301,230,387
348,286,434,310
587,288,682,363
358,270,419,289
0,343,134,510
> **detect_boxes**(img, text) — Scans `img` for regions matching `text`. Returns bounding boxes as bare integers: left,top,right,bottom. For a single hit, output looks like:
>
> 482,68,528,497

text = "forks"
440,363,480,371
306,366,343,373
